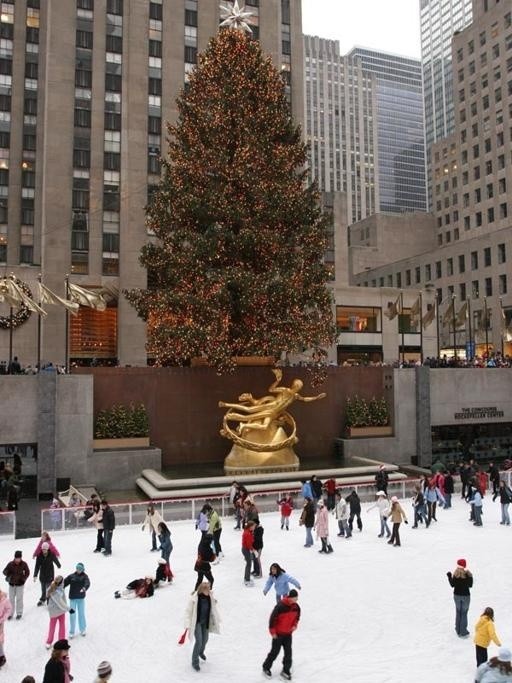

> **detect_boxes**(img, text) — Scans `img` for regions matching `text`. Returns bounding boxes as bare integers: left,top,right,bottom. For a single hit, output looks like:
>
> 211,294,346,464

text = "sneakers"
115,595,121,599
93,547,112,556
7,614,23,621
114,591,119,595
232,464,511,554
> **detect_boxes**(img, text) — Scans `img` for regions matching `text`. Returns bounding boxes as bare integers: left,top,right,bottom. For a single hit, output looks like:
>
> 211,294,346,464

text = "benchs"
58,484,92,527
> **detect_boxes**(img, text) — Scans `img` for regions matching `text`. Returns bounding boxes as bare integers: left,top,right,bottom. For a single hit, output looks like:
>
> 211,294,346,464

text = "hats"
289,589,298,597
53,639,71,651
146,574,154,579
158,558,167,565
15,550,22,557
41,542,50,550
97,660,113,677
457,559,466,570
76,563,85,571
497,648,512,662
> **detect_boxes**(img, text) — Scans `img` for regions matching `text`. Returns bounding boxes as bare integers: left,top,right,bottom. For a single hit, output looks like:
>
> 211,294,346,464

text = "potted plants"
92,399,153,450
341,393,394,437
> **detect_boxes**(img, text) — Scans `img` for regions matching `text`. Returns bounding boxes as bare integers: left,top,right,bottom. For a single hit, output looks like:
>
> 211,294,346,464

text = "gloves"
447,572,452,577
70,609,75,614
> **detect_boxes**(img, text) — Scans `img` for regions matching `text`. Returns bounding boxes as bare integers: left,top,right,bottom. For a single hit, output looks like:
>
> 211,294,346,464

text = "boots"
263,667,271,676
199,651,206,661
38,600,45,606
244,571,262,585
283,665,291,676
460,631,469,638
151,547,157,552
46,643,51,649
80,631,86,637
192,661,201,671
69,633,75,639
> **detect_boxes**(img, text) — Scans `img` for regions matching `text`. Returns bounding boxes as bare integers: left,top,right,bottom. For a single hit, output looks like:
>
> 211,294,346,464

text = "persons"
218,367,328,436
2,354,122,376
309,351,512,368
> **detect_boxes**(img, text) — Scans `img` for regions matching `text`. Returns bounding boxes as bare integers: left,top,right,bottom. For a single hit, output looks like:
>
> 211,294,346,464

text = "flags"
8,280,47,318
383,292,507,332
38,282,79,318
0,279,23,310
67,280,107,313
